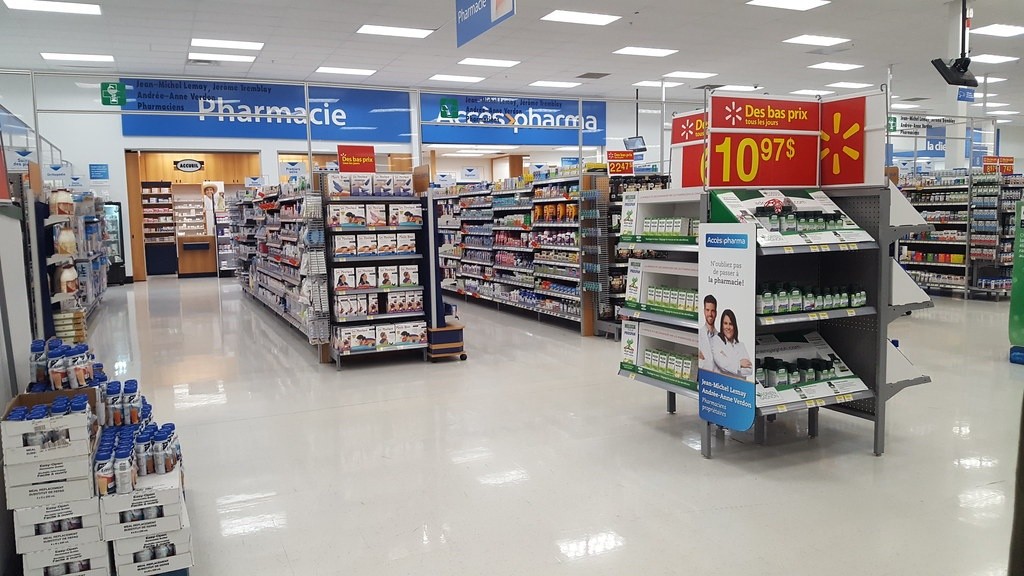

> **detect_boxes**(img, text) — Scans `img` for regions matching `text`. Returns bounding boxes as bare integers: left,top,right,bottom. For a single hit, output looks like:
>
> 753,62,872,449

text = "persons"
401,331,420,342
359,244,377,252
405,211,422,223
379,332,388,344
359,273,371,286
379,244,396,251
202,183,218,234
370,304,378,313
344,212,365,224
337,275,349,287
336,244,355,253
698,294,754,380
382,273,391,285
390,300,421,311
398,243,415,251
403,272,412,284
357,335,375,346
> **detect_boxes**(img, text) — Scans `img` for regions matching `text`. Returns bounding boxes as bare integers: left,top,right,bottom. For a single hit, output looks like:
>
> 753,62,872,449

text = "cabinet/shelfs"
141,181,177,274
897,164,1024,303
217,171,429,370
617,82,935,459
25,184,108,345
171,182,217,278
618,176,934,460
432,164,672,343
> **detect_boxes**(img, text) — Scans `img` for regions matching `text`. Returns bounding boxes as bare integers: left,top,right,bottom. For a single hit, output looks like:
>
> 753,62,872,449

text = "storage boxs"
323,171,966,424
0,386,194,576
